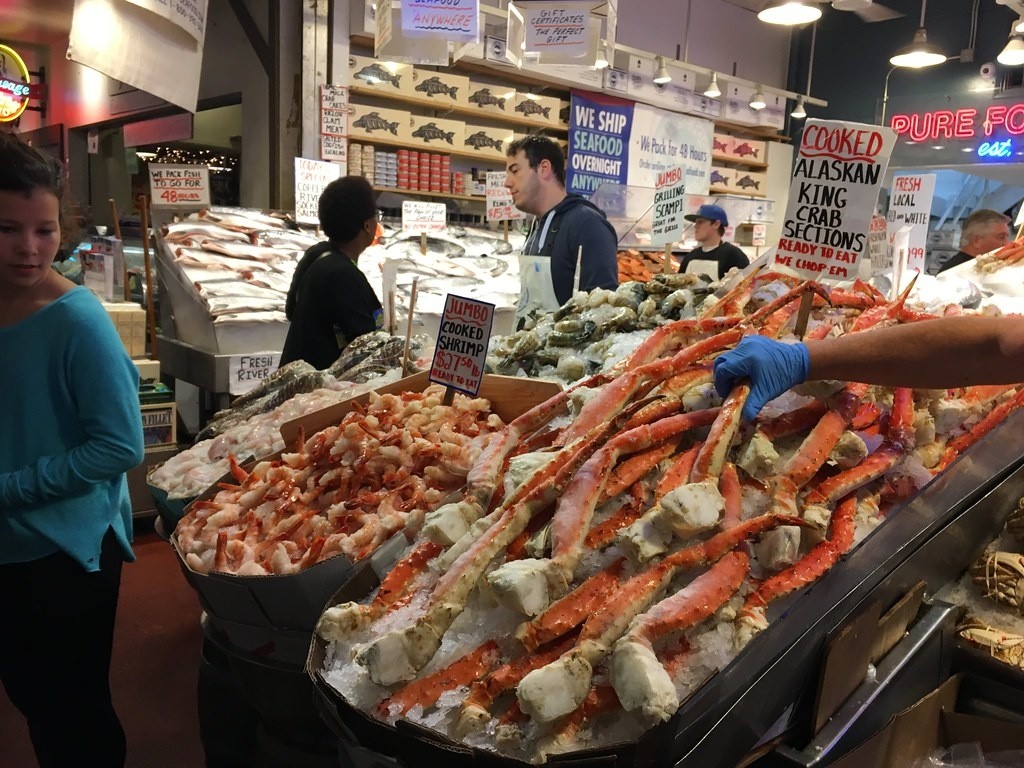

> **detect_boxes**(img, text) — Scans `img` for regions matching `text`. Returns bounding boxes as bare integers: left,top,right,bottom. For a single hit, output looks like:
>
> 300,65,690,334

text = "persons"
0,137,145,768
504,136,618,335
278,176,383,370
677,206,749,280
713,313,1024,420
939,209,1011,273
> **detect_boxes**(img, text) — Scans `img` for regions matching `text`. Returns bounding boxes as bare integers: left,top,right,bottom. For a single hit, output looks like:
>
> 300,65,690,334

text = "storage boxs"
486,35,788,130
77,236,178,447
711,134,767,194
349,55,571,159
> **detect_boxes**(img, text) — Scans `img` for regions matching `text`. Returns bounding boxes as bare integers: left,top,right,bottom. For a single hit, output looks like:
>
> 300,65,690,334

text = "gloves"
712,334,811,423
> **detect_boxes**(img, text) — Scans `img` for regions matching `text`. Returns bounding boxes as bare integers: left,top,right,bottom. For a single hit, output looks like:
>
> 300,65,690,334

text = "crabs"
314,262,1024,755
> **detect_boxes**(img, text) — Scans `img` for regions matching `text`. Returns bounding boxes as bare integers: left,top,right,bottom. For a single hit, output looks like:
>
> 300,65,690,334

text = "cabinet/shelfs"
348,37,793,262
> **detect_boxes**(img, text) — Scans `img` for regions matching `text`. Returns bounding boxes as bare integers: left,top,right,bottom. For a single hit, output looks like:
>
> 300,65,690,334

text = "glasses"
366,210,384,222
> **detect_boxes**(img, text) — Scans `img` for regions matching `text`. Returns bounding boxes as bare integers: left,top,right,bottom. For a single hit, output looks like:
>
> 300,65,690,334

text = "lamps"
889,2,947,68
750,85,766,109
791,95,807,118
653,56,671,83
758,0,822,25
595,41,608,69
704,72,721,97
997,13,1024,65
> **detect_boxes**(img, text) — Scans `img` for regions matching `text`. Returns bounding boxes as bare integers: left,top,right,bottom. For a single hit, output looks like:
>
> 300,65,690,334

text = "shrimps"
171,387,506,575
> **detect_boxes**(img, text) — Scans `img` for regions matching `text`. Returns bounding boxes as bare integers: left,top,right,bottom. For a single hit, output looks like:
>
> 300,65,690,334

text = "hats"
684,204,728,226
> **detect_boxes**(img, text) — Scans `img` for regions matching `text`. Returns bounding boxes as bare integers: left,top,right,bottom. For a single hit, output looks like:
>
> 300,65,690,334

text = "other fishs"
159,204,521,322
